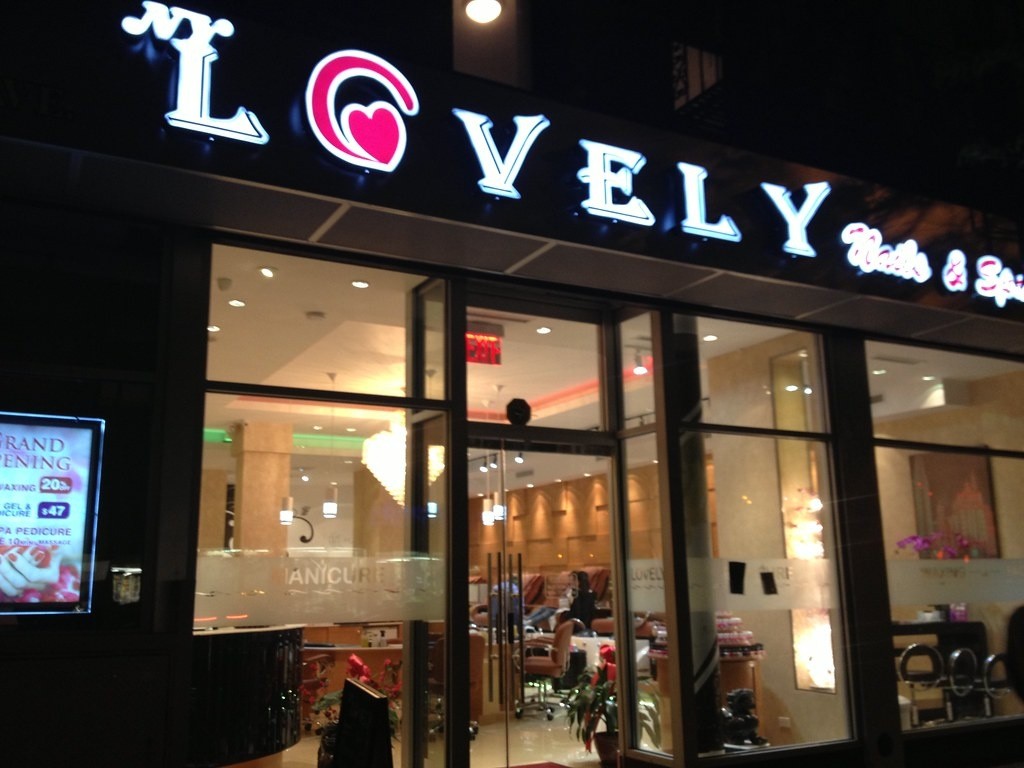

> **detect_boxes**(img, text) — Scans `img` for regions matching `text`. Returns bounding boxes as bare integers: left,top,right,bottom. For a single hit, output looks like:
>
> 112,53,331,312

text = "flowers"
633,348,647,375
894,532,989,560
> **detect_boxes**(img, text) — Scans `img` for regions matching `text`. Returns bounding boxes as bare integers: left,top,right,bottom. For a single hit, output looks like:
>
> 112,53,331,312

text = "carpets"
498,762,571,768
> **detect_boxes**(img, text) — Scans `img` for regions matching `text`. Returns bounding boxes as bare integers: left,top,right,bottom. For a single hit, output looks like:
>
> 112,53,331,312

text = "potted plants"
561,644,661,766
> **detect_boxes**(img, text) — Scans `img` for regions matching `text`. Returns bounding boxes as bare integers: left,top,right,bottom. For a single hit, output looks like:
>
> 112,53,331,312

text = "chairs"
378,567,667,742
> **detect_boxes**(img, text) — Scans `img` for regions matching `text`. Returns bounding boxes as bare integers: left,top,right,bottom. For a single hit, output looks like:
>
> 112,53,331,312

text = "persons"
524,571,597,634
491,573,525,644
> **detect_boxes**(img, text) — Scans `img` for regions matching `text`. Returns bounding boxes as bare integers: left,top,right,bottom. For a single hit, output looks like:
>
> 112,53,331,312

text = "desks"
647,650,767,756
890,619,990,730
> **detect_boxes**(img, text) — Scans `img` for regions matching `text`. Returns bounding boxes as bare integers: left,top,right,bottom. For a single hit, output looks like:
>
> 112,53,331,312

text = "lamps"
480,383,505,526
280,400,294,526
360,412,446,512
513,452,525,465
323,370,337,520
